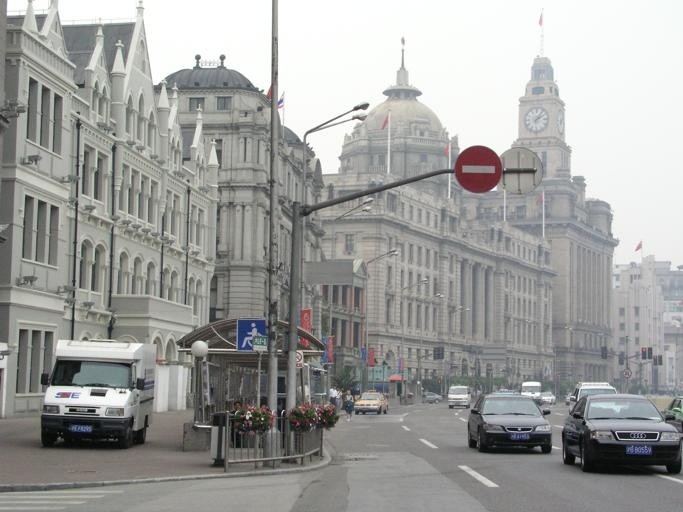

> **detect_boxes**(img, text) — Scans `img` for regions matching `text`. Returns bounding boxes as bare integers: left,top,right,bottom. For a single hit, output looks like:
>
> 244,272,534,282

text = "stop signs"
453,143,503,193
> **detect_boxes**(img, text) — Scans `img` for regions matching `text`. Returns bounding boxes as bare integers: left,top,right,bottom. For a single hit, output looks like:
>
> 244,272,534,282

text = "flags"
277,93,284,108
536,193,543,205
267,86,272,99
635,241,642,251
382,115,388,129
444,144,449,156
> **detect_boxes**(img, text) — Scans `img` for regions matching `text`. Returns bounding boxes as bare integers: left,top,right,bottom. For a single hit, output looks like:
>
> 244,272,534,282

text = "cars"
541,391,558,407
466,391,555,454
352,388,390,415
559,395,683,473
562,389,573,404
656,397,683,433
491,388,518,394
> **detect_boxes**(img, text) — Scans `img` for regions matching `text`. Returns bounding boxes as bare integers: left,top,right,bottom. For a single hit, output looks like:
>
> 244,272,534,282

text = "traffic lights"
642,347,662,365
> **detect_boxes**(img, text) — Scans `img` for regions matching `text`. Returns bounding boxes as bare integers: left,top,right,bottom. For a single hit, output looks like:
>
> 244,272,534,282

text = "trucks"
520,380,542,404
35,337,158,449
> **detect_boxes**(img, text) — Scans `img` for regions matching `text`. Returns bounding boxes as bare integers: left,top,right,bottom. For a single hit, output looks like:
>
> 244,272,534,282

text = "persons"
229,402,244,448
261,404,267,415
330,386,354,422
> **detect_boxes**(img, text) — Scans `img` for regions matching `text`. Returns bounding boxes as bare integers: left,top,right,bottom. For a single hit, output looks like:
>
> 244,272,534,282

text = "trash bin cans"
209,411,229,467
400,392,413,405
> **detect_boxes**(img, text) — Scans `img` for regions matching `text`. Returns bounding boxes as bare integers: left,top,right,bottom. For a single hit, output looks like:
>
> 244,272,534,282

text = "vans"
569,381,621,421
447,385,471,409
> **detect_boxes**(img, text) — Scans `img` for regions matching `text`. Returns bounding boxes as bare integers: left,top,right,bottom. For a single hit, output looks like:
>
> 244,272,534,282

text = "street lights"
361,249,471,404
297,99,373,355
191,338,208,429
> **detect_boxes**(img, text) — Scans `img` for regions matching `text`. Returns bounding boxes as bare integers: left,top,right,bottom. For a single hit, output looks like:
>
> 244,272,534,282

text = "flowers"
291,400,339,437
236,402,274,437
